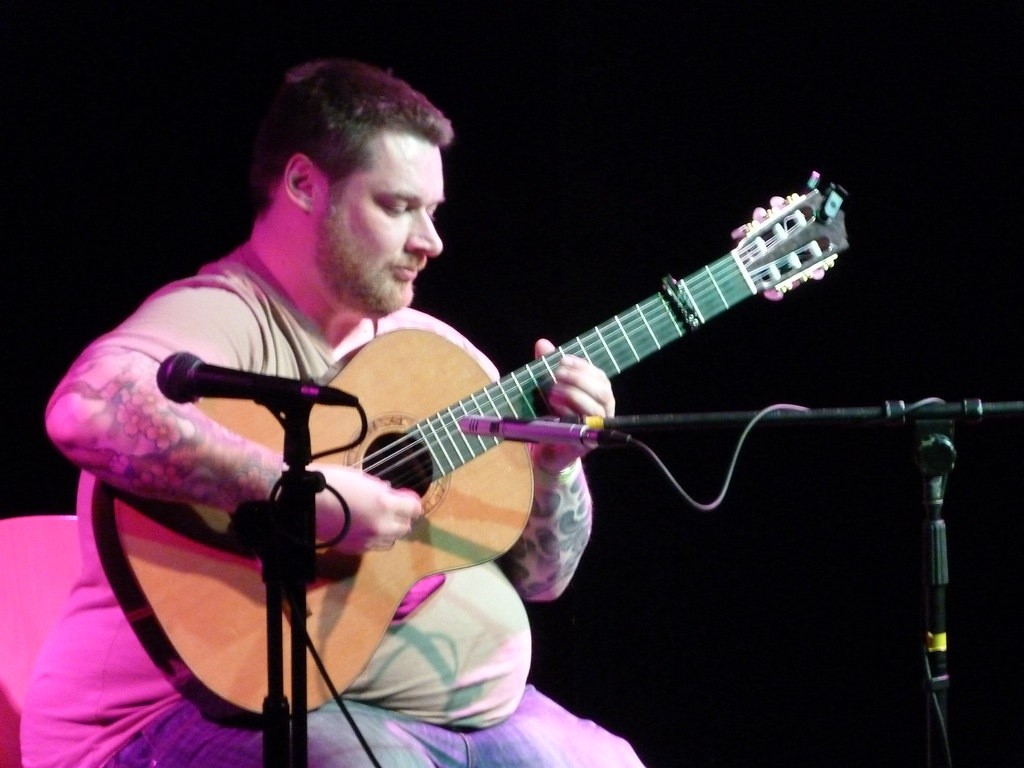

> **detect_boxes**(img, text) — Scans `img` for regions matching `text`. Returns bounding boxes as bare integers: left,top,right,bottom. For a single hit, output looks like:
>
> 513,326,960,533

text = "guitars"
89,169,849,725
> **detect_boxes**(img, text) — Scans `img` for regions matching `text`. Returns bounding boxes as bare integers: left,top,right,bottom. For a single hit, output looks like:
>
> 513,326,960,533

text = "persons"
42,59,647,767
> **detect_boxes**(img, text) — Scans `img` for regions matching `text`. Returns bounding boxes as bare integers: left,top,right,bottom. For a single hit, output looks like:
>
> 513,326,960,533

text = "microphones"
156,352,356,413
460,414,630,447
